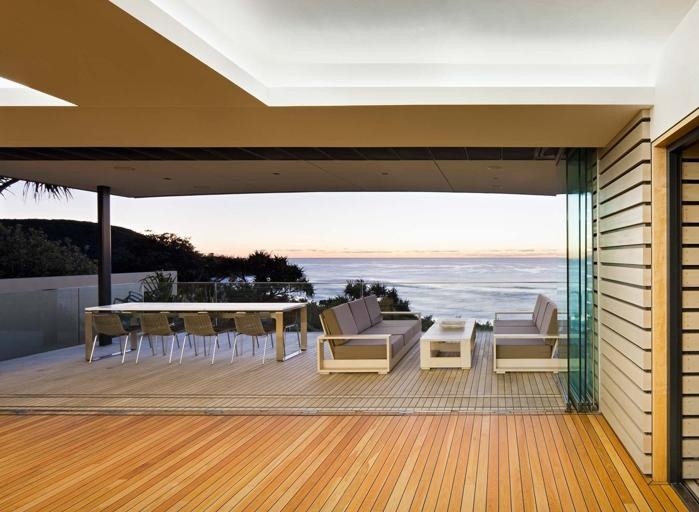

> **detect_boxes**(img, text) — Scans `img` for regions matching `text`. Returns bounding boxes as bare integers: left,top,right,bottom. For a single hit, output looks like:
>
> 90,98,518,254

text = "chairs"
88,310,299,366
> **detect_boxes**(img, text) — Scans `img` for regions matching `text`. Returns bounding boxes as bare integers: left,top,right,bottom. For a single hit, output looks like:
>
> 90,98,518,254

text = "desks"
83,301,309,362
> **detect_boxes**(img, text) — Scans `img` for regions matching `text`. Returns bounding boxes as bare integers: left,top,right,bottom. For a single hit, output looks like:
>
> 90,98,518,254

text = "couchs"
492,294,560,376
315,294,423,376
